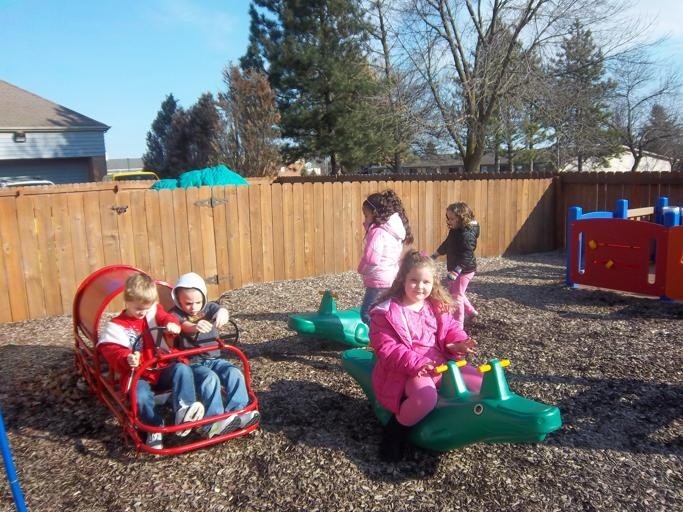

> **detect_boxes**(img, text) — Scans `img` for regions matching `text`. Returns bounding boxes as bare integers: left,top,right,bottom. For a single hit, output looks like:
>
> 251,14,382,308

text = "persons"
168,273,261,439
358,189,413,327
95,272,204,450
431,201,480,332
366,249,478,463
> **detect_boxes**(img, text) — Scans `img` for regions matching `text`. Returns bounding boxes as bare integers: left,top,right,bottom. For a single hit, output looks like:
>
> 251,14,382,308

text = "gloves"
446,269,459,281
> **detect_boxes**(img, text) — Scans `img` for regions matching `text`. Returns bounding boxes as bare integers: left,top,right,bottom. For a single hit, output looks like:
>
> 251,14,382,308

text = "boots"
377,414,413,463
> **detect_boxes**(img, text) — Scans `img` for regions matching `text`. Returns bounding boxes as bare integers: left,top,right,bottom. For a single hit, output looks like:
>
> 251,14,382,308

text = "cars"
1,175,56,188
100,170,160,181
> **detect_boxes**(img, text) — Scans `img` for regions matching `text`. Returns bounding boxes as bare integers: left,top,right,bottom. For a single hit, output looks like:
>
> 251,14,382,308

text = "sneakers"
239,410,260,429
146,431,164,449
175,398,206,436
204,413,241,440
